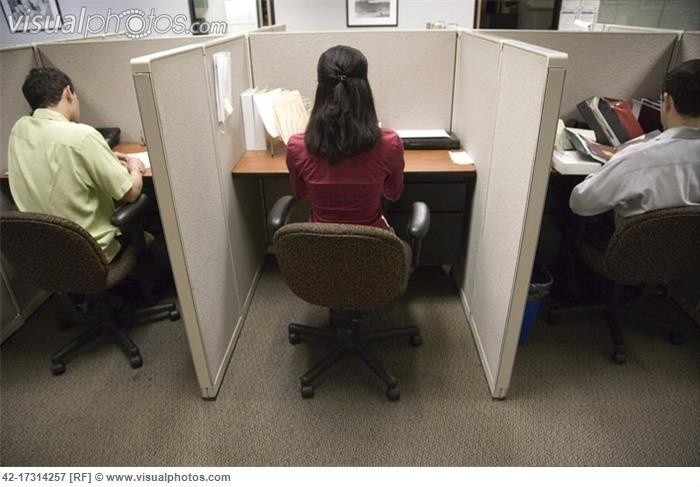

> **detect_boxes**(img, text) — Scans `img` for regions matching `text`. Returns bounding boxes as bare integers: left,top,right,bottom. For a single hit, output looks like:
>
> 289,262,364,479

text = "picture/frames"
347,0,399,30
1,0,64,33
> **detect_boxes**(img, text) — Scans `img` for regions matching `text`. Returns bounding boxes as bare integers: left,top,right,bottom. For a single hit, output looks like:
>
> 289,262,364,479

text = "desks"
1,143,155,201
551,150,601,181
232,146,474,177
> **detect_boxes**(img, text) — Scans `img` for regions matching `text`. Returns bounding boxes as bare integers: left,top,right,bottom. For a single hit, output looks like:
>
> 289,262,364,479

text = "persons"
557,58,700,268
284,44,406,233
7,67,175,301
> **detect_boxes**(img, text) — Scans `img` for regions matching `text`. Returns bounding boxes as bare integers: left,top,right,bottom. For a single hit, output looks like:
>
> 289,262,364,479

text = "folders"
240,86,267,150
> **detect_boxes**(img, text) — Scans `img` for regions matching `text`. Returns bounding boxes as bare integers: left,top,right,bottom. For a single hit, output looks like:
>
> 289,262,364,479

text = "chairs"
267,195,430,401
552,206,699,363
1,194,180,375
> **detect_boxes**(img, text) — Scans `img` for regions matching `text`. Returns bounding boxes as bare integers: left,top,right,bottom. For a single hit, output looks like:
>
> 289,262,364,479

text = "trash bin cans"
517,261,554,347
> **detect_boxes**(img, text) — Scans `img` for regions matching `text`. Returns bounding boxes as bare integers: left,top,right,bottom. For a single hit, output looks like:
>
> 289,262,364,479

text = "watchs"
132,165,147,175
